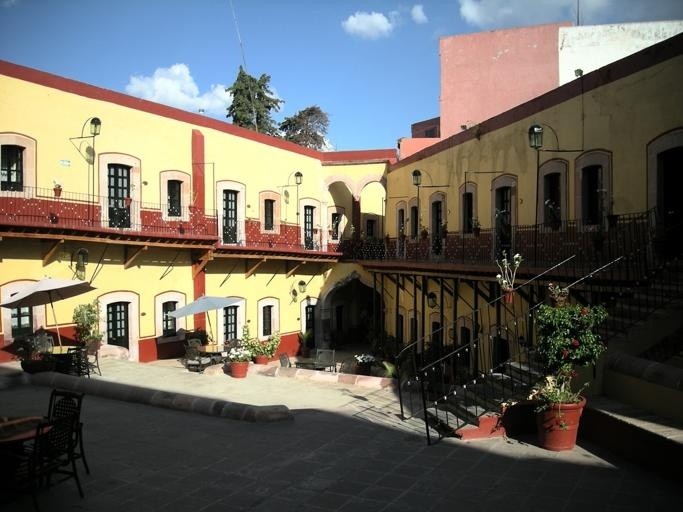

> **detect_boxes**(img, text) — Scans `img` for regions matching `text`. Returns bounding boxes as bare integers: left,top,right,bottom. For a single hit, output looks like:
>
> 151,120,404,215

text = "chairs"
184,339,237,374
0,388,91,512
33,335,101,379
279,348,335,372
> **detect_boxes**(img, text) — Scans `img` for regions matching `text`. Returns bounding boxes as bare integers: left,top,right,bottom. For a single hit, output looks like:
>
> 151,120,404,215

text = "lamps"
427,291,437,309
526,121,584,267
69,116,101,226
412,169,450,260
278,170,303,246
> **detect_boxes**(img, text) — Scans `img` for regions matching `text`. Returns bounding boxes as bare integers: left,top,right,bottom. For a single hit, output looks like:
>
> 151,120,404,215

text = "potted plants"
12,331,48,374
73,299,102,355
298,329,314,358
230,325,281,378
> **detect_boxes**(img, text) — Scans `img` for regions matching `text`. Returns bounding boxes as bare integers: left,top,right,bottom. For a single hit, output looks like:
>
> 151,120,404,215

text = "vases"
502,285,514,304
538,395,588,452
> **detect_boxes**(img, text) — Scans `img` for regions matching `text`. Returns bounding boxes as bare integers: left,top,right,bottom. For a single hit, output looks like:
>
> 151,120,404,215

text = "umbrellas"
167,292,245,344
0,278,99,350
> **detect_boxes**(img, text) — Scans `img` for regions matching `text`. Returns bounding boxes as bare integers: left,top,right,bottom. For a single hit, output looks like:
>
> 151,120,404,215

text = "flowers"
531,283,610,433
496,250,525,287
